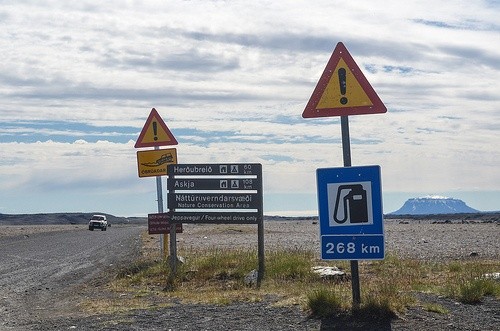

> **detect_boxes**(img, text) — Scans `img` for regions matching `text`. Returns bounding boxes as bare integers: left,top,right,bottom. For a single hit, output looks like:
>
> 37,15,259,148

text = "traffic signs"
166,163,262,226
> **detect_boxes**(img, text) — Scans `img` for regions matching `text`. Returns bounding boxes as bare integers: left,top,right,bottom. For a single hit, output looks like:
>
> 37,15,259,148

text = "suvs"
88,214,107,231
